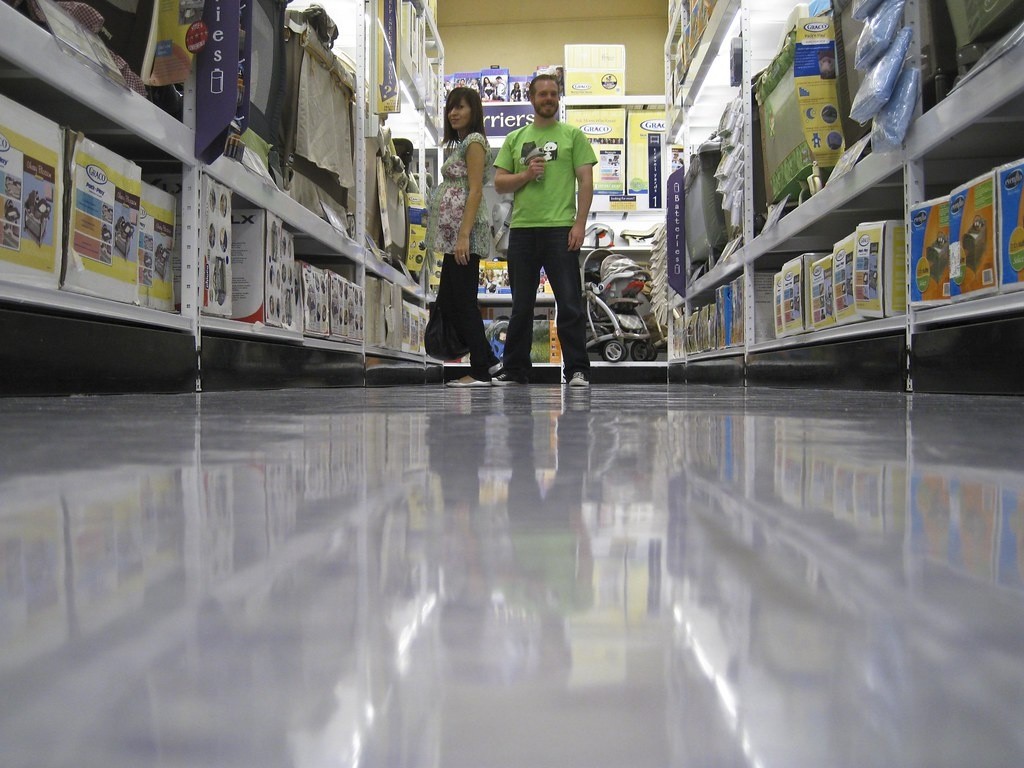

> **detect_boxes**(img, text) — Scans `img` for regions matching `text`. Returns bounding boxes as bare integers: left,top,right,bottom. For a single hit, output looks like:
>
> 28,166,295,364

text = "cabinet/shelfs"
0,0,444,395
661,0,1024,395
424,96,671,384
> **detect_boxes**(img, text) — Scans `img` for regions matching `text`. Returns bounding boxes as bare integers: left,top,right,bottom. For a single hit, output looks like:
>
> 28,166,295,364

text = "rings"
462,255,465,257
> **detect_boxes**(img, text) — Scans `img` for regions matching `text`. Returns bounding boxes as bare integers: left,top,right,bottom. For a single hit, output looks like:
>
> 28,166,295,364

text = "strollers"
578,249,668,363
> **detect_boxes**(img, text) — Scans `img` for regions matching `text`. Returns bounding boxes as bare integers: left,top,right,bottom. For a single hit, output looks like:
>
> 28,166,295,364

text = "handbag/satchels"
425,298,474,361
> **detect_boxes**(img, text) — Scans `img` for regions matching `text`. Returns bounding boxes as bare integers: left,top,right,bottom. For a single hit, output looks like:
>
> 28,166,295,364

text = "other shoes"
488,363,504,377
446,376,490,387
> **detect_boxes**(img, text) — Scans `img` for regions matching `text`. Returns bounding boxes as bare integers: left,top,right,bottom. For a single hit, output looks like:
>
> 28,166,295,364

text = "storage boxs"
235,210,430,356
564,44,627,95
667,156,1023,362
563,108,667,212
477,260,554,294
446,64,563,102
446,320,562,364
0,92,233,320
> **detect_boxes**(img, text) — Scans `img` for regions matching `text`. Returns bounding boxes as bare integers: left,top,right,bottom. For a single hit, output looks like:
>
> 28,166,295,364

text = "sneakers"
568,372,589,387
492,374,522,385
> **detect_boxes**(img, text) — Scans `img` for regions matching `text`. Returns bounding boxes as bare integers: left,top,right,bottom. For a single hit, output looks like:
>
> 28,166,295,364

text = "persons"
457,79,482,89
425,87,494,387
493,74,598,387
510,82,531,102
554,67,564,92
425,386,592,696
483,76,505,101
